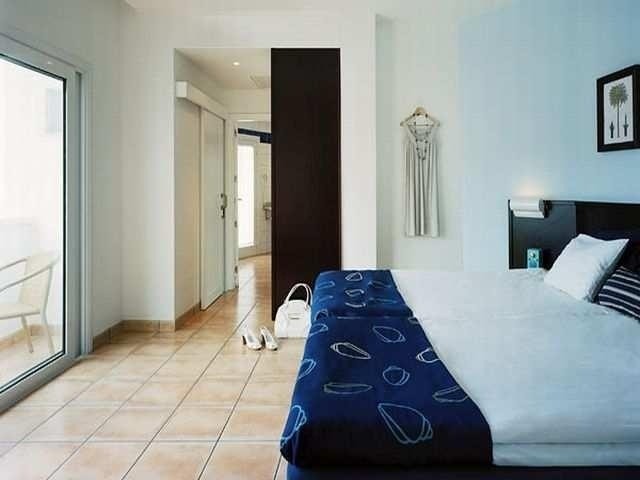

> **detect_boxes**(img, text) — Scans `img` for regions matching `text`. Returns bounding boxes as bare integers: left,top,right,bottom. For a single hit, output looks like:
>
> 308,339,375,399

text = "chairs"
0,249,63,354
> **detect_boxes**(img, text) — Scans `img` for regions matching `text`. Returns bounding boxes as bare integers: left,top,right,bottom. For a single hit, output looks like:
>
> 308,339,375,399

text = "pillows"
543,234,640,323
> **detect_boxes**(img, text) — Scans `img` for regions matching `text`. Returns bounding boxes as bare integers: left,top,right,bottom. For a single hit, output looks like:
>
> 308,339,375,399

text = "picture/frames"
596,64,640,153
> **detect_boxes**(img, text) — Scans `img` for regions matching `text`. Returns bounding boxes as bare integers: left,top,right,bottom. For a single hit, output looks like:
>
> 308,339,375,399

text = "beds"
311,268,640,321
280,318,640,479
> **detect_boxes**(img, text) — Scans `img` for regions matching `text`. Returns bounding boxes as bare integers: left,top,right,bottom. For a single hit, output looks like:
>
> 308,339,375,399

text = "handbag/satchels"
274,283,313,338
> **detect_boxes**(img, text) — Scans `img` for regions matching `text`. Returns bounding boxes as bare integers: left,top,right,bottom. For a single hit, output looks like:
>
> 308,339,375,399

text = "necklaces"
413,113,429,161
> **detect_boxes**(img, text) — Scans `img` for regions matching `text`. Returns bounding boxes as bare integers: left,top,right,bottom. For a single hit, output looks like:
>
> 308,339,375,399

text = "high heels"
241,326,278,351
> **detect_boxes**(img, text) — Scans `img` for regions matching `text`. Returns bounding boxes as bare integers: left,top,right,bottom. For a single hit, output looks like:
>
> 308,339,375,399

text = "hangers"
398,105,443,128
